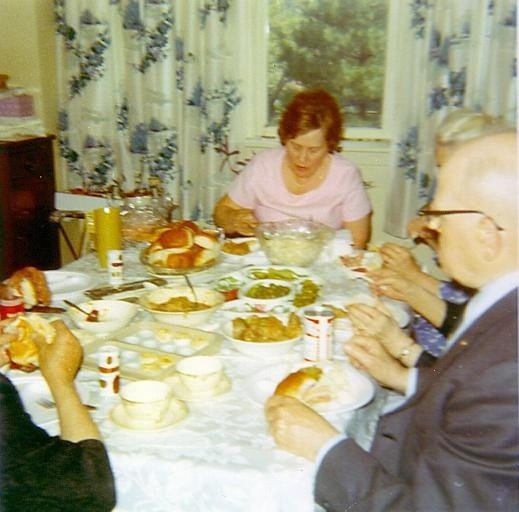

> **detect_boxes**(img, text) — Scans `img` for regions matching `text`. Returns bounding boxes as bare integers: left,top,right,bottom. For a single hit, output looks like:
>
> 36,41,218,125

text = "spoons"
182,270,199,306
64,300,103,322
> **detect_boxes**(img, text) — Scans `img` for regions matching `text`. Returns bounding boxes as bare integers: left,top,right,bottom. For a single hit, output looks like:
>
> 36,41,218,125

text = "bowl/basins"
220,315,305,358
138,284,225,326
254,220,336,268
67,299,140,333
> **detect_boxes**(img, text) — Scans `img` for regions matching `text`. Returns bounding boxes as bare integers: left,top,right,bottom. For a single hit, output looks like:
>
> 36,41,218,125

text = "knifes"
23,304,65,315
206,261,254,285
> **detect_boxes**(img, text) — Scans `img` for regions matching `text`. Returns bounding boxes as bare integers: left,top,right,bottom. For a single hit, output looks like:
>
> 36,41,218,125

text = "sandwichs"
7,266,54,310
6,313,58,373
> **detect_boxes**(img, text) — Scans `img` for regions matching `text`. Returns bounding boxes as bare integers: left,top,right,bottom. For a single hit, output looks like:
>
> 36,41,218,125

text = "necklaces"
286,154,331,187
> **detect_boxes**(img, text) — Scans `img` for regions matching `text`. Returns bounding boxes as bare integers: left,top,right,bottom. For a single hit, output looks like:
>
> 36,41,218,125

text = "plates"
138,251,224,275
25,378,92,427
219,237,260,258
212,264,325,316
108,399,189,433
297,297,411,333
336,249,381,278
80,319,224,382
163,373,233,400
43,269,98,300
242,359,377,417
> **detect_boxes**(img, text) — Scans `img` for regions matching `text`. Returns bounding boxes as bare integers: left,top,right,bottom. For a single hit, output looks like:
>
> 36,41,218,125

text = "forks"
35,397,96,411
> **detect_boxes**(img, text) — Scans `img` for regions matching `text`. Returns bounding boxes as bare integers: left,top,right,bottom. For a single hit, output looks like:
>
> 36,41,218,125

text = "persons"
264,133,519,512
380,110,510,348
212,89,372,248
0,319,117,511
345,268,469,371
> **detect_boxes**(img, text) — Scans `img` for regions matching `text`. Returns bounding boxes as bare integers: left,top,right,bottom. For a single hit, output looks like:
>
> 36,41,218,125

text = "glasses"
417,204,504,231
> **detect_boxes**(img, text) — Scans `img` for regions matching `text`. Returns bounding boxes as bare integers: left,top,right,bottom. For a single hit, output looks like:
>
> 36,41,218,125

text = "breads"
273,366,336,407
144,220,221,269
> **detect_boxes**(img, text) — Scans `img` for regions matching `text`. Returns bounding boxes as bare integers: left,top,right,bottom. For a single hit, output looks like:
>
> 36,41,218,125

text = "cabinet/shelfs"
0,132,63,283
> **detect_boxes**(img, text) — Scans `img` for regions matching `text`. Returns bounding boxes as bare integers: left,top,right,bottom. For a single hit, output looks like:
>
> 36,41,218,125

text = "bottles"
97,345,120,395
107,250,123,285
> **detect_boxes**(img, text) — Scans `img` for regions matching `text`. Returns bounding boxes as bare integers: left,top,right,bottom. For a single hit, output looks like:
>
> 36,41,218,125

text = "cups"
174,355,222,392
119,380,173,421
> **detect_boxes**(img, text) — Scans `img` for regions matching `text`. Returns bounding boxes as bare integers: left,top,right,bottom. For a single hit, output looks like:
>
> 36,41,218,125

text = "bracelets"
395,340,418,362
